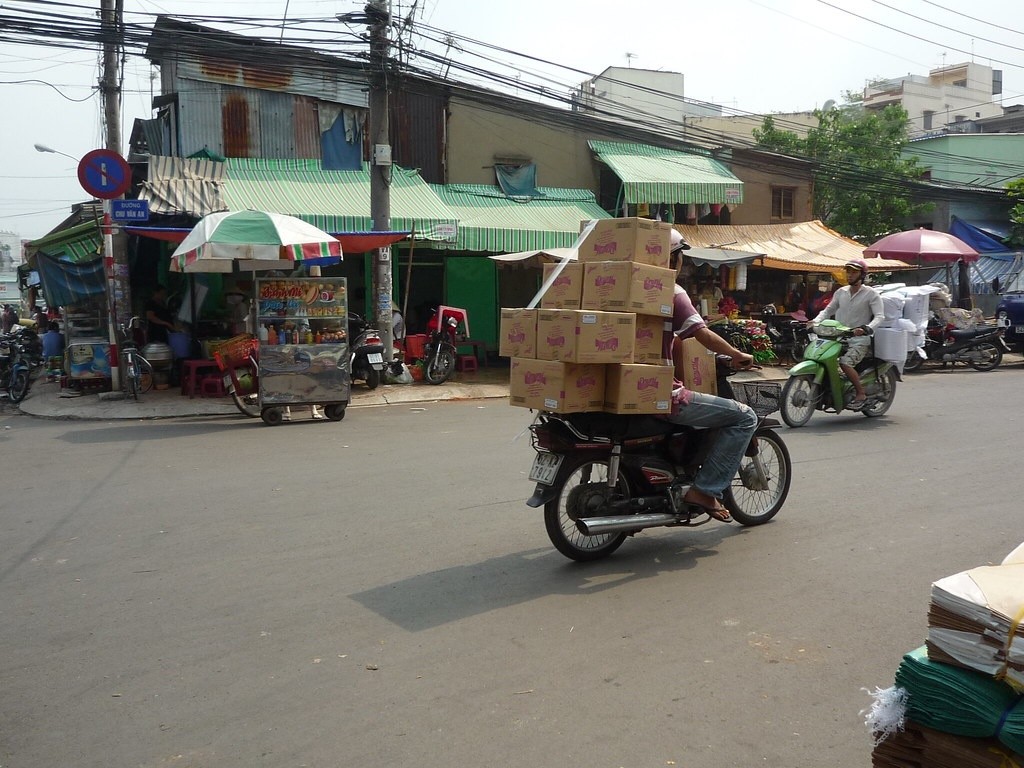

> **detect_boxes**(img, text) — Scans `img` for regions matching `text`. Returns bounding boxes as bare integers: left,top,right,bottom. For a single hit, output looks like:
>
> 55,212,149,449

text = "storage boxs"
498,218,719,416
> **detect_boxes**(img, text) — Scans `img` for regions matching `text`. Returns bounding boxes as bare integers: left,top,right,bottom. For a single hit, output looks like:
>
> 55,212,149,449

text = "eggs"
324,332,346,340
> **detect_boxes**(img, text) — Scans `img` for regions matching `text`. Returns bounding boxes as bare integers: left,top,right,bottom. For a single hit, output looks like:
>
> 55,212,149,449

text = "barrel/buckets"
405,334,432,357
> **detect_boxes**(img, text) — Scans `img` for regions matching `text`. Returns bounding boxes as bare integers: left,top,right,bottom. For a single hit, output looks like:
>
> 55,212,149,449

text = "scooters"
347,310,389,388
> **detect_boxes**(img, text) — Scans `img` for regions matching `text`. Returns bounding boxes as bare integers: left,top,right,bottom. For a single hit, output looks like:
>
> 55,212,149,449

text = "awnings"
98,144,460,390
406,163,615,262
914,215,1024,295
671,220,924,287
587,140,744,218
16,215,107,308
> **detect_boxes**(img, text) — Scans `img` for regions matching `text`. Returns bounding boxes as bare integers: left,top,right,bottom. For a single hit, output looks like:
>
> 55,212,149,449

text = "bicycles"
119,316,155,401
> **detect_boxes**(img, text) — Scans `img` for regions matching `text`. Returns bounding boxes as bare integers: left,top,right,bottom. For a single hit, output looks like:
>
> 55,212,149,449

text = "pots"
138,341,177,372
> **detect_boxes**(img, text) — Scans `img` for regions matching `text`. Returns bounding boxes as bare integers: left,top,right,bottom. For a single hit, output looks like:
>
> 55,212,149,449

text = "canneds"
307,307,328,315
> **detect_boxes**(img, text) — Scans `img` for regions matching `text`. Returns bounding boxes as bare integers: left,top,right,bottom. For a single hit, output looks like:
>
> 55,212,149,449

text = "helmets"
669,227,691,253
843,259,869,274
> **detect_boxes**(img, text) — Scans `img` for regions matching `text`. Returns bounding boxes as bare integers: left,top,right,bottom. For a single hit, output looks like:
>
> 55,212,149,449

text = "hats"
31,306,39,310
8,306,16,311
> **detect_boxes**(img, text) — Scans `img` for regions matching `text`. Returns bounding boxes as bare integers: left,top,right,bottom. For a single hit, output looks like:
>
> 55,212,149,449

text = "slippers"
683,494,734,523
845,398,870,410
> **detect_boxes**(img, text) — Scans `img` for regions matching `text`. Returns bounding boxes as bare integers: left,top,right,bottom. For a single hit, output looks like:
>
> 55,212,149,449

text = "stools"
201,376,225,400
459,355,477,377
484,348,503,372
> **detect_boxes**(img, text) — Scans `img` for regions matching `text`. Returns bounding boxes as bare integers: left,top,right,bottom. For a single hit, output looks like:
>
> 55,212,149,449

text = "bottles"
308,306,337,316
260,323,268,345
279,324,312,344
46,356,63,382
260,281,300,299
168,331,192,357
316,331,321,344
269,325,276,345
407,363,422,381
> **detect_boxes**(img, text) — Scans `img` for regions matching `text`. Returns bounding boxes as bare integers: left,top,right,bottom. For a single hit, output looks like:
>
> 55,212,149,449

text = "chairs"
406,333,425,364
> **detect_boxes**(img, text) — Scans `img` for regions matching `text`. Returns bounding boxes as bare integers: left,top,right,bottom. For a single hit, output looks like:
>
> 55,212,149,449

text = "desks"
451,340,486,372
424,306,471,344
180,359,229,400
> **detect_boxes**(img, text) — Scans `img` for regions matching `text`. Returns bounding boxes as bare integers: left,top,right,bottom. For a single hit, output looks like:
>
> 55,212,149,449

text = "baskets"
731,381,782,415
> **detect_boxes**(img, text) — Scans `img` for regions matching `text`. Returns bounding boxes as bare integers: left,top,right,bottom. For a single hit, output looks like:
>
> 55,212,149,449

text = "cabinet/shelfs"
252,276,350,345
63,304,102,351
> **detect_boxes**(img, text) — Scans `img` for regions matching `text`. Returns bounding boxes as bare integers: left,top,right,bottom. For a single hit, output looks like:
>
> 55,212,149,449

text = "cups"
310,265,320,276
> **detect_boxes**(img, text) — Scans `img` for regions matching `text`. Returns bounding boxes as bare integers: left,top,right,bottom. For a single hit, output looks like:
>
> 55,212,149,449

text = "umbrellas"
168,208,344,339
862,227,980,286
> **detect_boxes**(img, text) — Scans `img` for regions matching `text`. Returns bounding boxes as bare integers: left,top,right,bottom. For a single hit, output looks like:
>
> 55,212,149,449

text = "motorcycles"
902,315,1011,372
0,326,34,403
526,350,794,560
779,320,902,427
425,307,465,386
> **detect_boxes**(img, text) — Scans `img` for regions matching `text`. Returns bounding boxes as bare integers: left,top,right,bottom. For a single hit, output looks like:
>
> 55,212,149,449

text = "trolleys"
256,327,368,425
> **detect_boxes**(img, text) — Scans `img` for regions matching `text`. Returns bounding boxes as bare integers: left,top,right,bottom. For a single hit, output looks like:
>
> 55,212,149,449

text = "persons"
144,284,177,343
3,304,66,365
392,300,407,342
223,288,247,335
650,227,758,523
806,261,885,410
